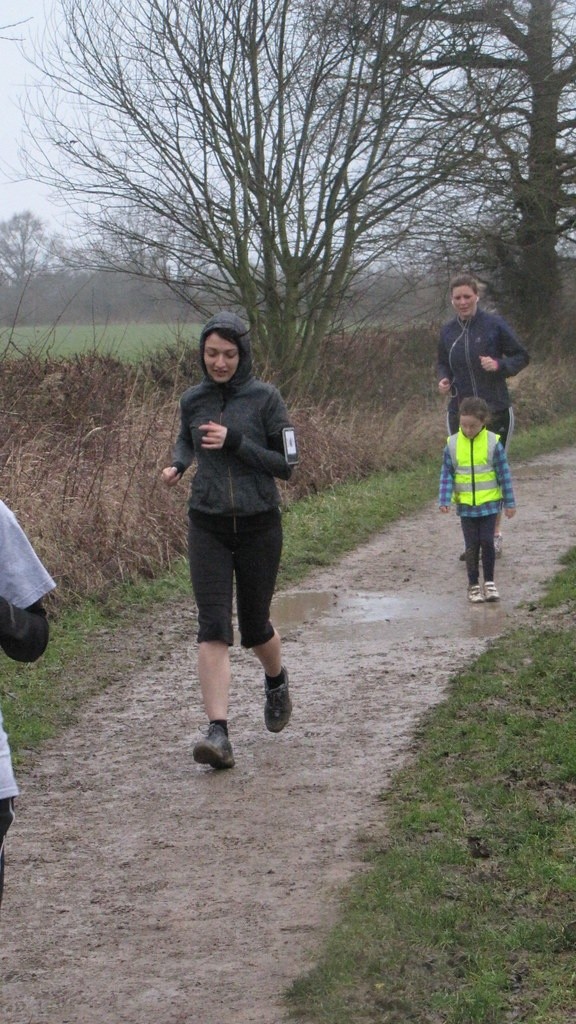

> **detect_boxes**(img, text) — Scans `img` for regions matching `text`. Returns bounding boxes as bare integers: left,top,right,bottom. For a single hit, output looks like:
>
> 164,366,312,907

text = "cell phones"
282,427,299,465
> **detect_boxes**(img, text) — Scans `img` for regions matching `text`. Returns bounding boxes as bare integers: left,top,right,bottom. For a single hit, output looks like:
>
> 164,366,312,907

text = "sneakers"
193,726,235,769
484,581,500,600
465,584,482,601
264,666,292,733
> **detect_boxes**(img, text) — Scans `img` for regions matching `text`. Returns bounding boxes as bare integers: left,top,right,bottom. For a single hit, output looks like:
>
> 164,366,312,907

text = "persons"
0,501,58,901
439,397,517,602
161,310,299,767
436,274,530,561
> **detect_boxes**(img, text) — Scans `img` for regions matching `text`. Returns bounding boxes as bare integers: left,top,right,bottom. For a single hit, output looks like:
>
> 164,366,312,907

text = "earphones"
476,297,479,303
451,300,453,304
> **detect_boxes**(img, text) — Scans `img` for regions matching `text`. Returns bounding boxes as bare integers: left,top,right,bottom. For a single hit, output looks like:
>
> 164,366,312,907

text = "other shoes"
494,535,504,558
459,549,483,561
